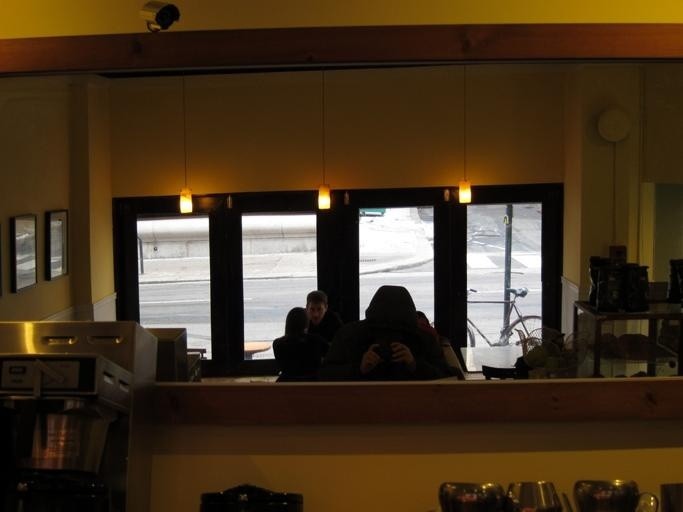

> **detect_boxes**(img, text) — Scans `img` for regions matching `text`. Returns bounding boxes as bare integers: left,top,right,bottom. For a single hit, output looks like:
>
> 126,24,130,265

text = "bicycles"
466,288,542,346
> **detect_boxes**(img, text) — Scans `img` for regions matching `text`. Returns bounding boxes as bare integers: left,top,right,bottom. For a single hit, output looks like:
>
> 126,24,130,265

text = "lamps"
178,70,195,215
458,63,473,204
318,71,331,211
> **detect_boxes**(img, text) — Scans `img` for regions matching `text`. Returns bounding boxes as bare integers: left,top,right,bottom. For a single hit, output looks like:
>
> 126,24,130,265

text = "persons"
324,284,451,381
273,307,330,369
304,291,344,339
416,309,441,341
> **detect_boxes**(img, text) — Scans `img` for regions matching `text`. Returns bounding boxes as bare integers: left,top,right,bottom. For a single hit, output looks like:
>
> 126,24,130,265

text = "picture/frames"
45,209,69,281
12,214,38,293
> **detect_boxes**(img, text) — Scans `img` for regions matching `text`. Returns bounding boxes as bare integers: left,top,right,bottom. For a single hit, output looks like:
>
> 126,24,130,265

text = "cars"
15,254,36,278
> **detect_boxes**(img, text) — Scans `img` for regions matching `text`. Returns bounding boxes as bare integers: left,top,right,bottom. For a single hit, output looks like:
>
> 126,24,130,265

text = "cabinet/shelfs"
573,300,683,377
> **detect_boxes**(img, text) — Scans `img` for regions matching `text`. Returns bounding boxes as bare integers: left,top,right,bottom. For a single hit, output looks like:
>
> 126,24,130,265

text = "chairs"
482,365,516,379
